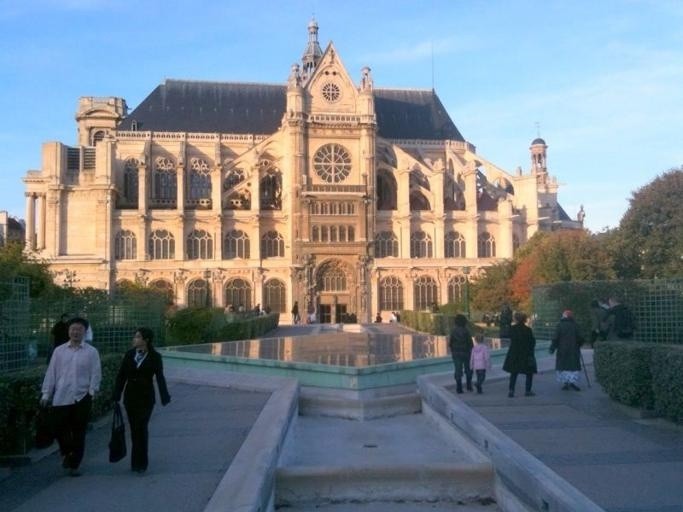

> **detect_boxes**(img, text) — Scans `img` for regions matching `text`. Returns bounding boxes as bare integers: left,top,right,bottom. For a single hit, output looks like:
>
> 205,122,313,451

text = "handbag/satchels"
108,403,128,463
31,403,57,450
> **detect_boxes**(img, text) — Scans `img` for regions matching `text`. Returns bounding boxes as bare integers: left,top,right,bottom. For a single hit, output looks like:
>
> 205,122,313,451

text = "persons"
389,312,397,323
449,313,474,394
375,312,381,323
588,301,608,349
291,301,300,324
109,326,169,474
502,311,538,397
395,312,400,322
78,313,92,346
546,309,585,390
225,300,270,317
603,297,634,341
306,301,315,324
468,332,490,395
37,316,102,469
48,312,70,348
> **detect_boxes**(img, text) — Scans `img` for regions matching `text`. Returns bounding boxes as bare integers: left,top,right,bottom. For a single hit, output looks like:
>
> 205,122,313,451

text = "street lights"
203,267,212,308
463,266,471,321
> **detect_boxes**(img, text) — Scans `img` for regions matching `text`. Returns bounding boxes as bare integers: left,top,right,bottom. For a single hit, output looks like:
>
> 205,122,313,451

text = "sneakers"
456,383,582,397
